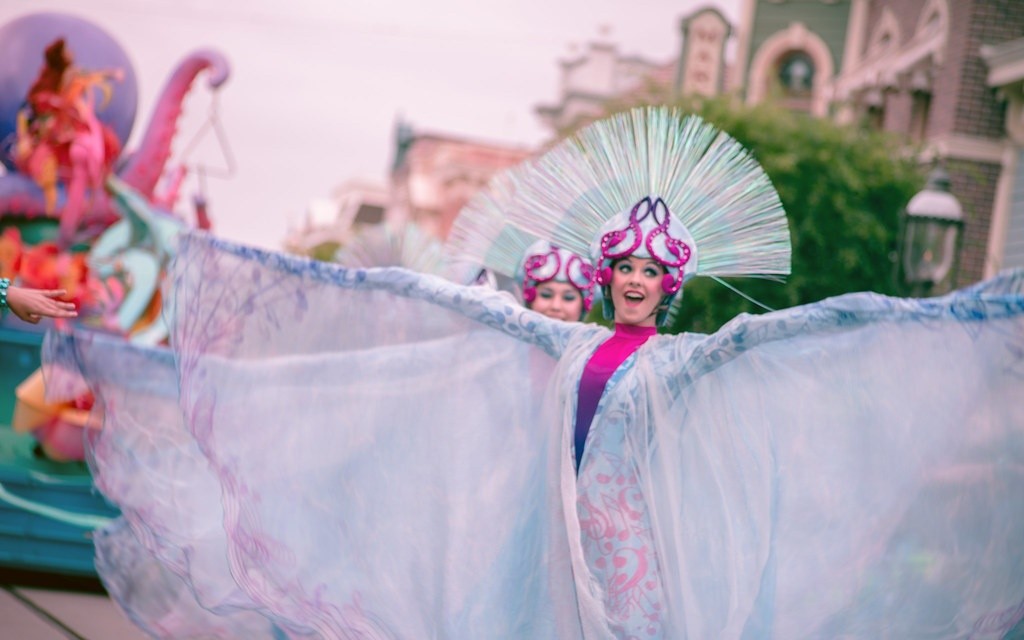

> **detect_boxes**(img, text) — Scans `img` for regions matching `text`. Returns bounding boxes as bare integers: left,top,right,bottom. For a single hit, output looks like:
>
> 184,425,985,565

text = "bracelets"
1,278,11,307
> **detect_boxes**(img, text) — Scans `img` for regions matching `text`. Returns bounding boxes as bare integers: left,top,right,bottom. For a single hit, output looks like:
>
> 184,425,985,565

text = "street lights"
898,166,964,299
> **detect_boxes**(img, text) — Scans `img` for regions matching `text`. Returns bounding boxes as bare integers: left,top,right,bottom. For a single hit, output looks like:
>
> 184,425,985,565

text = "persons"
0,105,1024,640
